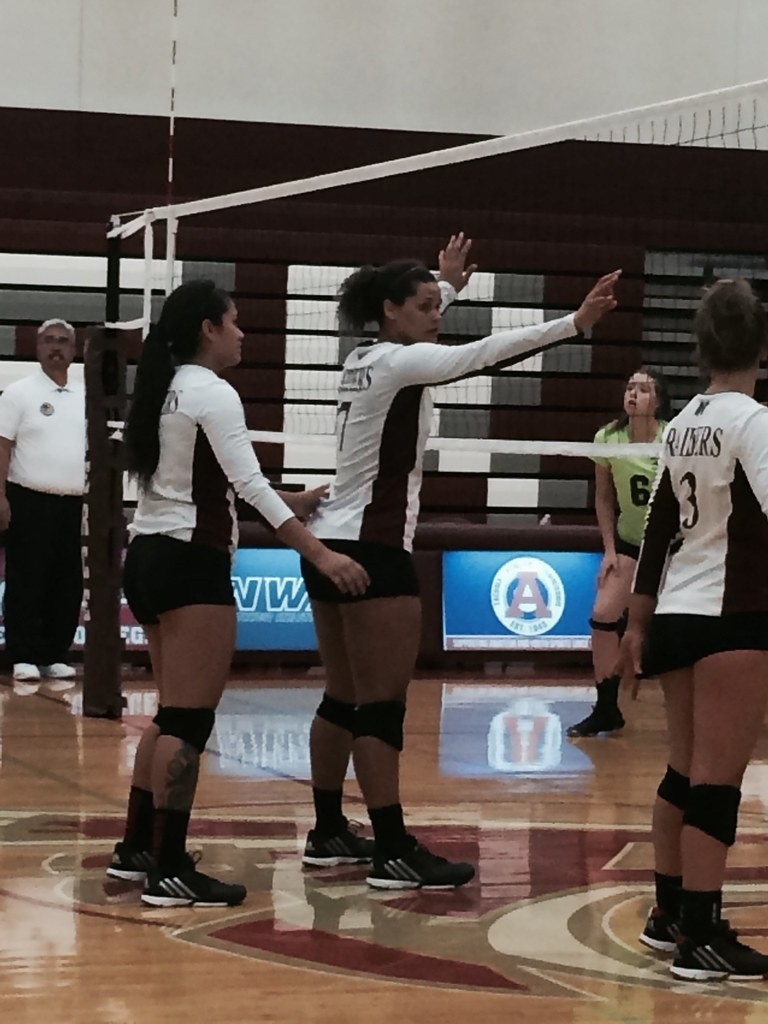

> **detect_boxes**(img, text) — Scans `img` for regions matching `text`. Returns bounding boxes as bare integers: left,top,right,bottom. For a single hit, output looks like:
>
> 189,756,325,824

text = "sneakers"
140,850,247,907
566,705,625,737
302,819,375,868
106,842,147,882
37,663,76,678
366,844,475,890
638,906,679,952
13,663,41,682
670,919,768,982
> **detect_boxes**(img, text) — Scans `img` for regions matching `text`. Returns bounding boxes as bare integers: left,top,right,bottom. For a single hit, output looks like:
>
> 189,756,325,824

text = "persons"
104,278,371,908
294,229,622,892
0,317,87,682
612,279,768,981
564,363,673,737
